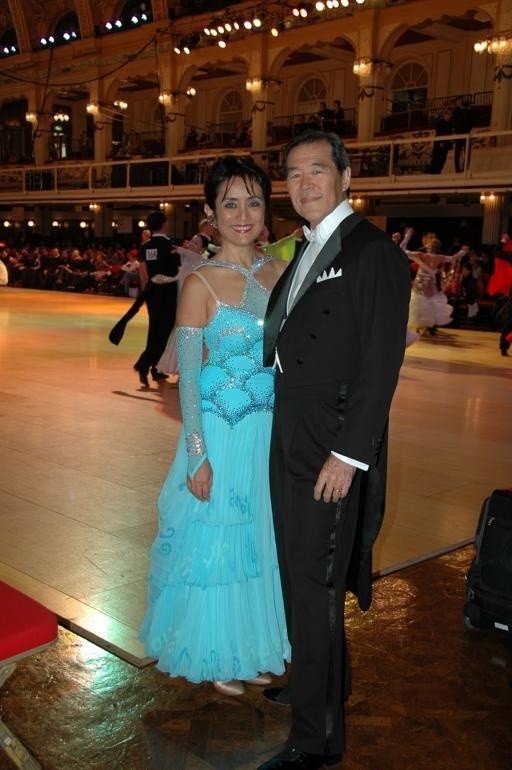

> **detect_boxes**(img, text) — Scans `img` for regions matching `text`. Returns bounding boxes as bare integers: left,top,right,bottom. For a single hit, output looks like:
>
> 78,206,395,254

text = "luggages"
461,490,511,637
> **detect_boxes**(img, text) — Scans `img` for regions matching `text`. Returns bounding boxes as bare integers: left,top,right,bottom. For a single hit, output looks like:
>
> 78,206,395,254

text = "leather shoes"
255,744,343,769
241,670,273,686
211,675,245,695
262,685,291,707
152,375,169,381
134,365,149,386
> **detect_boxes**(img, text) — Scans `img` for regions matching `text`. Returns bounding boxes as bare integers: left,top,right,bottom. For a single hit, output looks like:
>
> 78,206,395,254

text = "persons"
138,156,293,697
257,129,412,770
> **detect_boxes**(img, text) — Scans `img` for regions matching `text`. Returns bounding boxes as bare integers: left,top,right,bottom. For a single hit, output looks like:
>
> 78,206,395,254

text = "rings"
334,489,341,492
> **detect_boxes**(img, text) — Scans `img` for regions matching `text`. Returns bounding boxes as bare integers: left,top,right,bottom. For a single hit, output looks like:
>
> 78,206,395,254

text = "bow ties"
303,224,324,245
